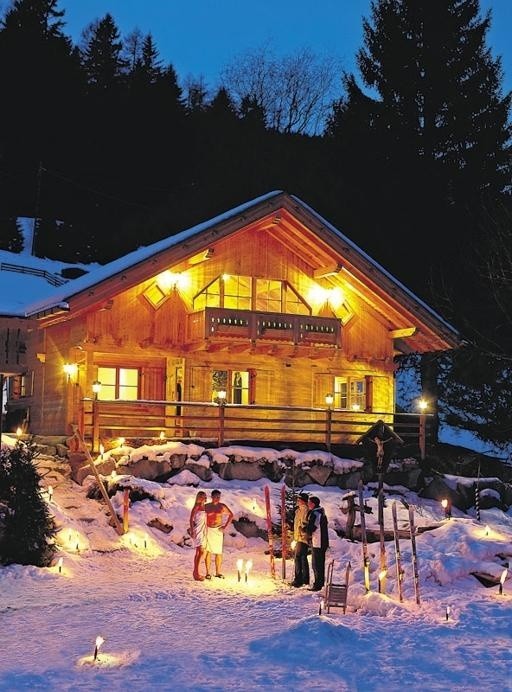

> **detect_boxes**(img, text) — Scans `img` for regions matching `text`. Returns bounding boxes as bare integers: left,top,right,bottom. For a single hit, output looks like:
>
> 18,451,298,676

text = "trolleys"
319,558,351,616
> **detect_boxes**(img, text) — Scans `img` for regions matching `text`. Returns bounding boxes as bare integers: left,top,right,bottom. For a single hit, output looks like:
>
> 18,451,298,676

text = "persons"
186,491,209,583
299,496,330,593
285,492,311,588
205,488,234,581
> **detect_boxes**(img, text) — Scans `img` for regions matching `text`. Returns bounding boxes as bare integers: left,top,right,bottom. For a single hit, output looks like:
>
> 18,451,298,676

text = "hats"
296,493,309,503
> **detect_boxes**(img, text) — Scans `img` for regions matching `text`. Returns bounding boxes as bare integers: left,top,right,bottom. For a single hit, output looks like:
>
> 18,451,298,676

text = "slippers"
206,574,211,579
215,575,224,579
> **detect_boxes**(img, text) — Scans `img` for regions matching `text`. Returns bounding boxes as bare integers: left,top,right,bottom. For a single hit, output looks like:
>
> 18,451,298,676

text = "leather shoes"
307,586,321,591
288,582,299,587
